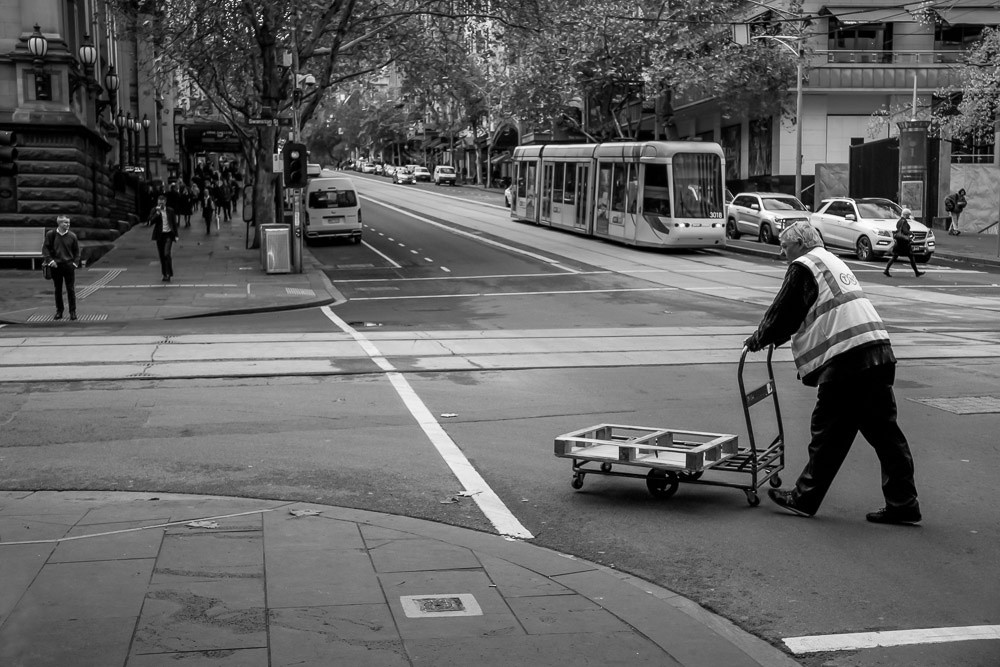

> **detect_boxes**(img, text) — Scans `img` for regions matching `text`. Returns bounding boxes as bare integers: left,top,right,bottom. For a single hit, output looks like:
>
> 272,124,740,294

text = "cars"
504,185,512,207
357,161,431,184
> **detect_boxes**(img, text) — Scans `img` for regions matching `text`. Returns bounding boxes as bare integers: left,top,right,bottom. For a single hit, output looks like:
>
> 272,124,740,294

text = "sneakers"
769,488,811,517
866,507,923,524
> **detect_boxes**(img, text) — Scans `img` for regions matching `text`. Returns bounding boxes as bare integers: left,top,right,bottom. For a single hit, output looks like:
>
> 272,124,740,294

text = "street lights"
750,35,802,200
116,107,142,173
142,113,151,183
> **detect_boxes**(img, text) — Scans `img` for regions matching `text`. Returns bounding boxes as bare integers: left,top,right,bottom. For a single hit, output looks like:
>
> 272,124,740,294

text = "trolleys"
572,343,785,506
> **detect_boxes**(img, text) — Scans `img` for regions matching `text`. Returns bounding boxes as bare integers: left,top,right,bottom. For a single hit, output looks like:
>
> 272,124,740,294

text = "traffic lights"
283,140,307,188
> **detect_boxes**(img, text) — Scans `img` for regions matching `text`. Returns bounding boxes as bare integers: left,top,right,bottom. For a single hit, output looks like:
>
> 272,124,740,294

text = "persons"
742,221,923,524
944,189,968,236
149,196,179,282
42,215,80,320
882,208,925,278
143,159,242,234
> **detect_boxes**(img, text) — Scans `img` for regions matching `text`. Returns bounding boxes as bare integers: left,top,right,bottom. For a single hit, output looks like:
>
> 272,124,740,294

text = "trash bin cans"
260,223,292,273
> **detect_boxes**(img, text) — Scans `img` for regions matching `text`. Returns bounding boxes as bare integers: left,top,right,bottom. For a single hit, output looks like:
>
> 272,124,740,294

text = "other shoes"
55,313,62,319
916,271,926,277
162,275,170,282
883,271,892,277
948,230,960,236
70,312,76,320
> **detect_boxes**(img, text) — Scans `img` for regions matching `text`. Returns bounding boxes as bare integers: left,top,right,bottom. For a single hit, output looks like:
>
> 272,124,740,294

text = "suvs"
688,186,813,243
810,197,936,262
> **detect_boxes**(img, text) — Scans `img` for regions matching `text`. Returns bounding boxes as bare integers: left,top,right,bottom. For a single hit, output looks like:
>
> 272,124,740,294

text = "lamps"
68,33,97,104
27,22,52,101
95,63,119,123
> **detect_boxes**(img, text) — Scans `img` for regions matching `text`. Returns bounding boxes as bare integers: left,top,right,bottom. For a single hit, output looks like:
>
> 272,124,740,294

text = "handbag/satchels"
894,220,911,242
41,262,52,280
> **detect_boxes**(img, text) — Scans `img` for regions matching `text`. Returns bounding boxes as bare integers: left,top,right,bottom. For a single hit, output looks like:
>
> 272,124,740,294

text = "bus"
511,140,727,248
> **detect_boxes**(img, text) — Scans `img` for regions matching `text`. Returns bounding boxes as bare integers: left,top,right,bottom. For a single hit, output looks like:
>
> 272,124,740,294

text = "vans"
302,176,362,243
434,165,456,186
